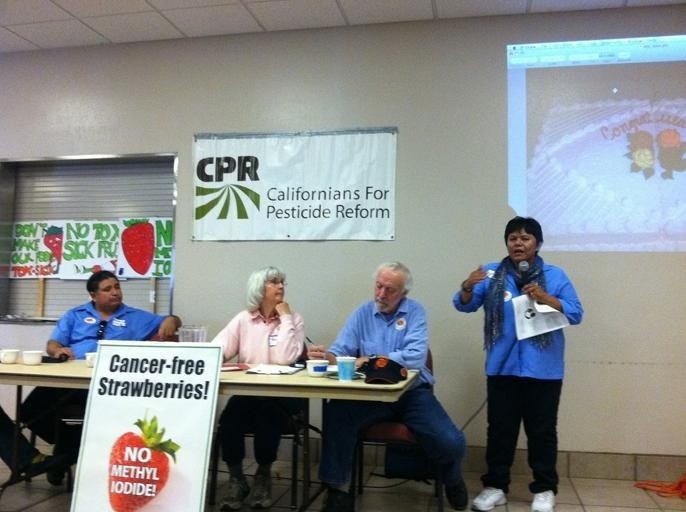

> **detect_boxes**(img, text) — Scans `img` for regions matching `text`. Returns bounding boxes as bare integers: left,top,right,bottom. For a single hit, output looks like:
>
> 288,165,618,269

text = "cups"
0,349,20,365
176,324,208,344
334,355,357,381
84,352,97,367
307,358,328,379
21,350,44,365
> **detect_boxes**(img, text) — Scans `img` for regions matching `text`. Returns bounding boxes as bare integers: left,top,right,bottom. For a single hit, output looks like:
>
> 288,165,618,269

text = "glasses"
96,321,107,339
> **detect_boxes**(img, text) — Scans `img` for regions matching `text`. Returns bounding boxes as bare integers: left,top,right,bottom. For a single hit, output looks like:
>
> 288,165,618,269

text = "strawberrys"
107,416,179,512
43,226,63,265
121,218,155,275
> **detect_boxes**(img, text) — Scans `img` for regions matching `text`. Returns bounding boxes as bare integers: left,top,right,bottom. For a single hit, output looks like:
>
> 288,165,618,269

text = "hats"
365,358,407,384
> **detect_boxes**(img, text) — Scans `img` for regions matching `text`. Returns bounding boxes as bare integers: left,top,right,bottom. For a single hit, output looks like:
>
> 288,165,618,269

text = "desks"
1,350,421,512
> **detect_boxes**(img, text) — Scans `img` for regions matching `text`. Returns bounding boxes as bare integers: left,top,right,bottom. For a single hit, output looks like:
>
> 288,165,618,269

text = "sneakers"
471,487,507,511
531,489,556,511
445,478,467,511
249,473,273,508
24,455,58,475
219,477,250,511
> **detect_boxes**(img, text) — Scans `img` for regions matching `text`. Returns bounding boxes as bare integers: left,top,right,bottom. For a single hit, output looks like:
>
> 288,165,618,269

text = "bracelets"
461,280,472,293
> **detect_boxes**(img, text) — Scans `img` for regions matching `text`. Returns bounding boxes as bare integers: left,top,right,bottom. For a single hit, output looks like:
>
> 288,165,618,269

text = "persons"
452,215,584,512
19,269,182,486
308,260,468,511
207,265,304,511
0,405,66,480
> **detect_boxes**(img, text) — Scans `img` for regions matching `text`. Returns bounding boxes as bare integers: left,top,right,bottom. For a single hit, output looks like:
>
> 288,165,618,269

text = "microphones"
518,260,529,286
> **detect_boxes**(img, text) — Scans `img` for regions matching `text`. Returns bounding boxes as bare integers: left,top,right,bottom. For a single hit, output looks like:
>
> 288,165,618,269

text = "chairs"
24,413,83,493
208,340,315,512
346,346,436,512
